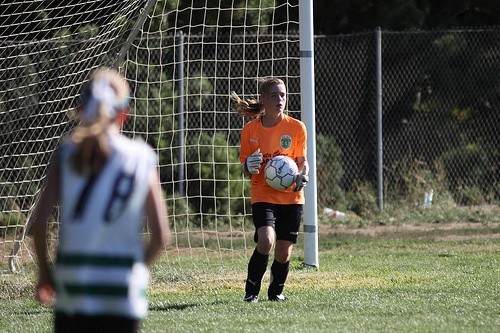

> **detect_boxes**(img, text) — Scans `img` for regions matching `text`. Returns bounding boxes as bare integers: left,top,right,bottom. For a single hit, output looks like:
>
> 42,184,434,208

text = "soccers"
262,154,299,190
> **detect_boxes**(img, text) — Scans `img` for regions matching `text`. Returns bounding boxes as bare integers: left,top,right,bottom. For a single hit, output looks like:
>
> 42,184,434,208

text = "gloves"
294,159,309,193
243,149,264,175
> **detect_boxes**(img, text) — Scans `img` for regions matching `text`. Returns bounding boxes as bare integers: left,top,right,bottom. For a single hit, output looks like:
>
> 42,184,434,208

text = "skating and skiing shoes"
244,293,258,302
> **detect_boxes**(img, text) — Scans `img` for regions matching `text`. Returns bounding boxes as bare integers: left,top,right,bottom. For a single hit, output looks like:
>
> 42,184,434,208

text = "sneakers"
268,295,287,302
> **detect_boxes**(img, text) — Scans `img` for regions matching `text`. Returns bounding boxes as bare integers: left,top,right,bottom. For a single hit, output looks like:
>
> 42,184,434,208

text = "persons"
229,78,310,303
31,67,171,333
